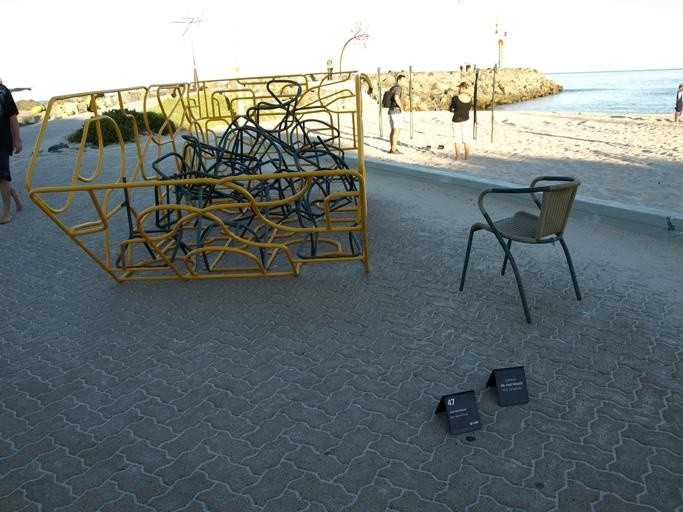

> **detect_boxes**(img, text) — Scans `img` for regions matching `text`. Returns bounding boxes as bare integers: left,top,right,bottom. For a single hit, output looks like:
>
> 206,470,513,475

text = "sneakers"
390,149,404,154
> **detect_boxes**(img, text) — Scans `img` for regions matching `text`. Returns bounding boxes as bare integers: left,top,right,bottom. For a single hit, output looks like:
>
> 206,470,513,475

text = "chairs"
459,176,582,323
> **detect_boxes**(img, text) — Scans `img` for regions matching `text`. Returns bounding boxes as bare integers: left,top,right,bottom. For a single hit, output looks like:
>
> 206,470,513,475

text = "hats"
456,81,469,89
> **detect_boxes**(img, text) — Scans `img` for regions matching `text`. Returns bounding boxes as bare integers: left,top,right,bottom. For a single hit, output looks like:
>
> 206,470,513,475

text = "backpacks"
675,91,683,112
382,85,399,108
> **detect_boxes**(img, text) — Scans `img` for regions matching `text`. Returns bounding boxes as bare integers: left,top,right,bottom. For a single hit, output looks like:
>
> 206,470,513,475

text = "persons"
0,80,24,224
447,82,473,161
387,75,408,156
673,84,683,123
